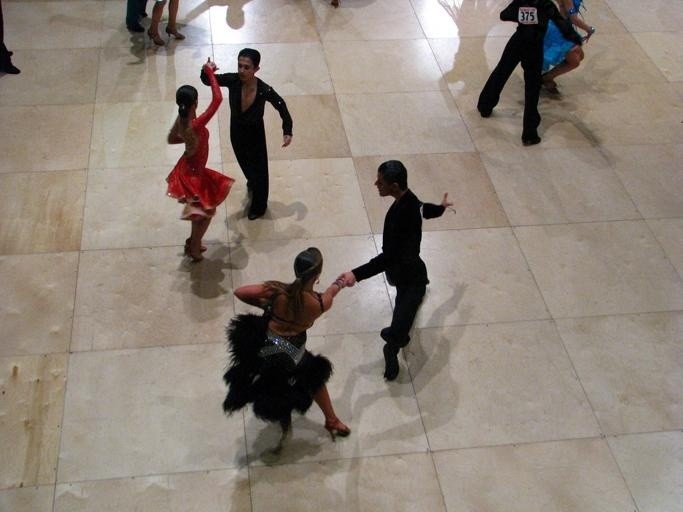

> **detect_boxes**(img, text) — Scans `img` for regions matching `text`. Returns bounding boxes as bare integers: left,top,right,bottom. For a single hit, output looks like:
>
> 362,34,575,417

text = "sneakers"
126,23,144,32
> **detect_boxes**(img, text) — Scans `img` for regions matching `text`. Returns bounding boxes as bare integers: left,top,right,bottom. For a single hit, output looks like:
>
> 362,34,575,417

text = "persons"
201,48,294,220
0,0,21,75
541,0,595,96
164,63,234,263
478,0,583,144
340,160,457,382
222,247,351,456
147,1,184,46
126,0,148,33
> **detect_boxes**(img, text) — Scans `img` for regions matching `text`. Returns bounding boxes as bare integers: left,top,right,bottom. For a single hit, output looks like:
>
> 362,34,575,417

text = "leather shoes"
1,65,20,75
247,205,260,220
523,137,541,144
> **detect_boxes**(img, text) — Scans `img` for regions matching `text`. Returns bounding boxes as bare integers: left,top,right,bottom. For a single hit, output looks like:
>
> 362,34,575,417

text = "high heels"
183,237,207,262
541,72,559,95
147,28,165,46
165,25,185,40
324,416,350,442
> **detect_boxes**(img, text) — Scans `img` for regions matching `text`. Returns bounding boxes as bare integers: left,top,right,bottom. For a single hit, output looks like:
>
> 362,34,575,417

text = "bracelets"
591,25,594,32
331,278,345,288
